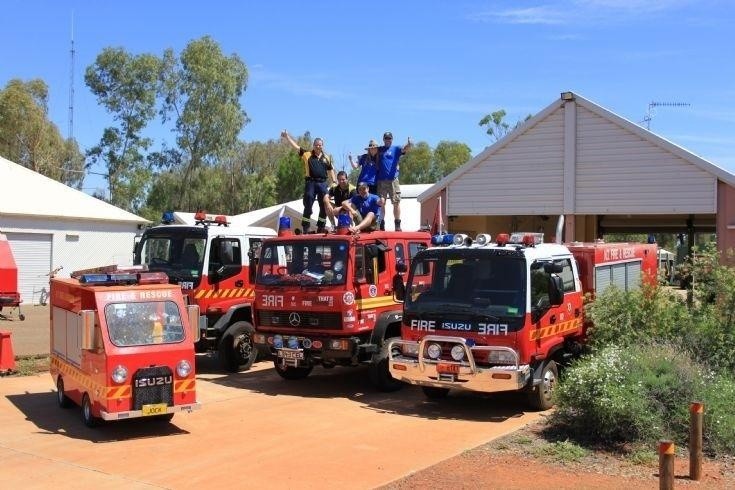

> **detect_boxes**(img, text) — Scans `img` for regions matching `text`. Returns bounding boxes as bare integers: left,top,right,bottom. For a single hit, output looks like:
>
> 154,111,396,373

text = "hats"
384,132,393,139
364,140,381,150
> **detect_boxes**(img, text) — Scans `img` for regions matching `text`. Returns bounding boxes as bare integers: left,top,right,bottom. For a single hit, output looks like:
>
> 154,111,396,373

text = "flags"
429,198,441,237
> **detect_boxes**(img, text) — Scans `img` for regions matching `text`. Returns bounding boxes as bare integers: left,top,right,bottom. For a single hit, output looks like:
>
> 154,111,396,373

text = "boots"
301,216,328,234
378,218,387,232
394,218,403,232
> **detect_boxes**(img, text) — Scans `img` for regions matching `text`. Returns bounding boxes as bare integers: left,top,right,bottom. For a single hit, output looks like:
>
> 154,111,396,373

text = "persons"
347,138,380,194
340,181,386,234
324,172,358,235
280,128,337,234
374,129,410,231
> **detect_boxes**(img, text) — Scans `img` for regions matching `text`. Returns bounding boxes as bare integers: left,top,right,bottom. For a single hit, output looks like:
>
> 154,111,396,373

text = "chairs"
180,244,200,266
444,263,477,299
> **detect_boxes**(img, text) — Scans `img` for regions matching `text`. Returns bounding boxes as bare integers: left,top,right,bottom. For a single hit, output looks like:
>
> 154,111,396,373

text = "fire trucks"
50,266,201,429
